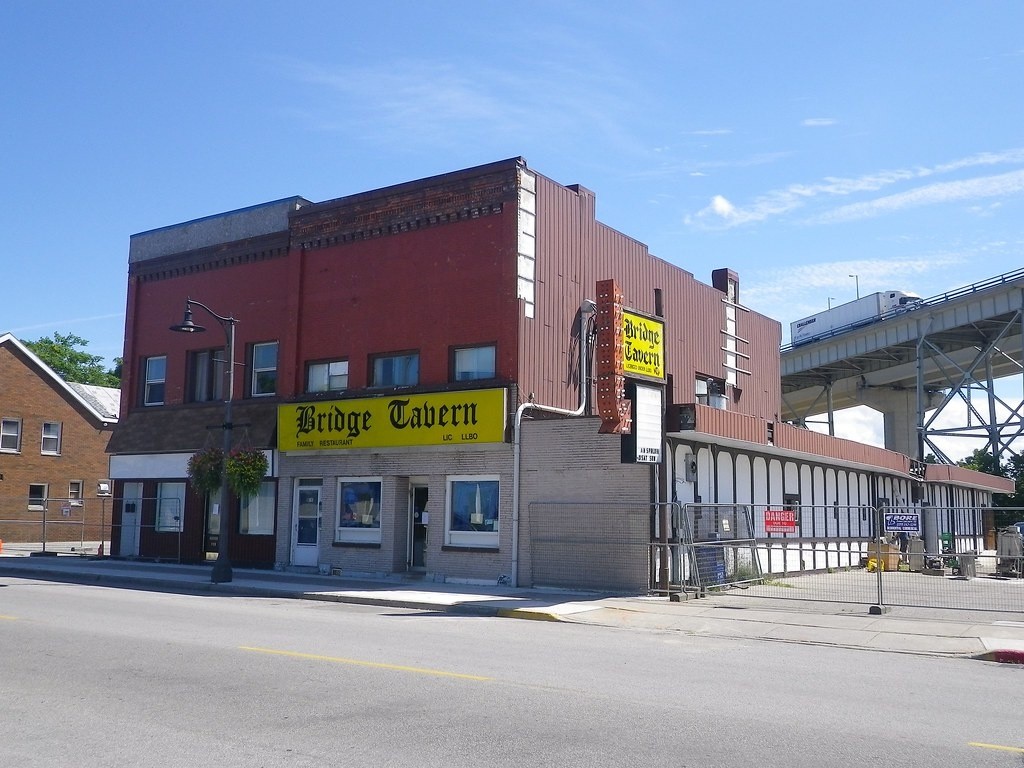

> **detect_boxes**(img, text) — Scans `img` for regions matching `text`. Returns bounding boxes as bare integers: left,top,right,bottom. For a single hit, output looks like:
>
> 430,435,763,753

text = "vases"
318,563,333,575
332,568,343,576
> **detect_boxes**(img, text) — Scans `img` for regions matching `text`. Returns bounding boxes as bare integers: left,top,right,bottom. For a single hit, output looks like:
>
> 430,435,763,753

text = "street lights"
169,297,240,582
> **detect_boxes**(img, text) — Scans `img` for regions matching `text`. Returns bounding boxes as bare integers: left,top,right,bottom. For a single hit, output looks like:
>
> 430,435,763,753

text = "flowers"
186,430,225,496
222,428,271,498
